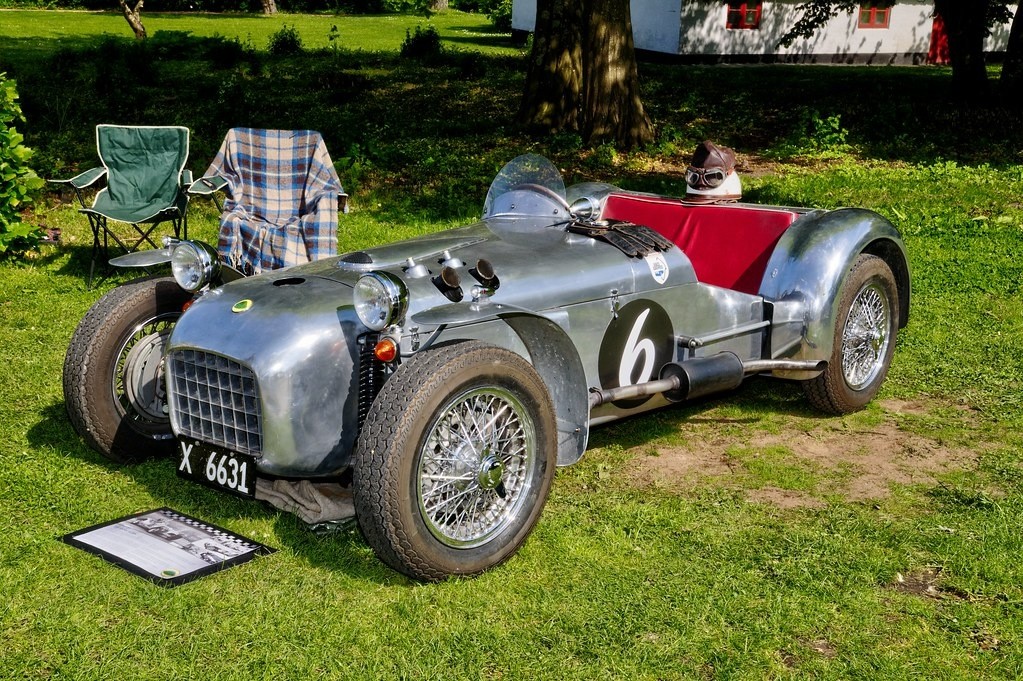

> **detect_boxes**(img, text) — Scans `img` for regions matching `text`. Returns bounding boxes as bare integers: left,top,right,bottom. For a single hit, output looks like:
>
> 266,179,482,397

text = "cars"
62,154,913,585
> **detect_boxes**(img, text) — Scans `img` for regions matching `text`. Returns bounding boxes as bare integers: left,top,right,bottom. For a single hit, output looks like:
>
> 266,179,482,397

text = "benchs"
596,192,799,297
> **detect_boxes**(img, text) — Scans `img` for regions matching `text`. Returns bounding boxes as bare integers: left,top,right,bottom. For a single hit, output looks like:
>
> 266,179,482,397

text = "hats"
680,139,742,203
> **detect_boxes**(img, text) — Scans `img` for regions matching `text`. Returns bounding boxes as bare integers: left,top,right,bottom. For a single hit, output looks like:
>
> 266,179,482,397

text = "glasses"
685,169,725,187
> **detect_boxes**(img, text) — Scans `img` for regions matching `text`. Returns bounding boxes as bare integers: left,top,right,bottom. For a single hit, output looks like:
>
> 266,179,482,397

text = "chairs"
48,124,349,291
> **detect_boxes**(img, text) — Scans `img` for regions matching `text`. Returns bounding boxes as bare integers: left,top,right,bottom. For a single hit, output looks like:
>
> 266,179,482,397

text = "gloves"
566,219,674,257
